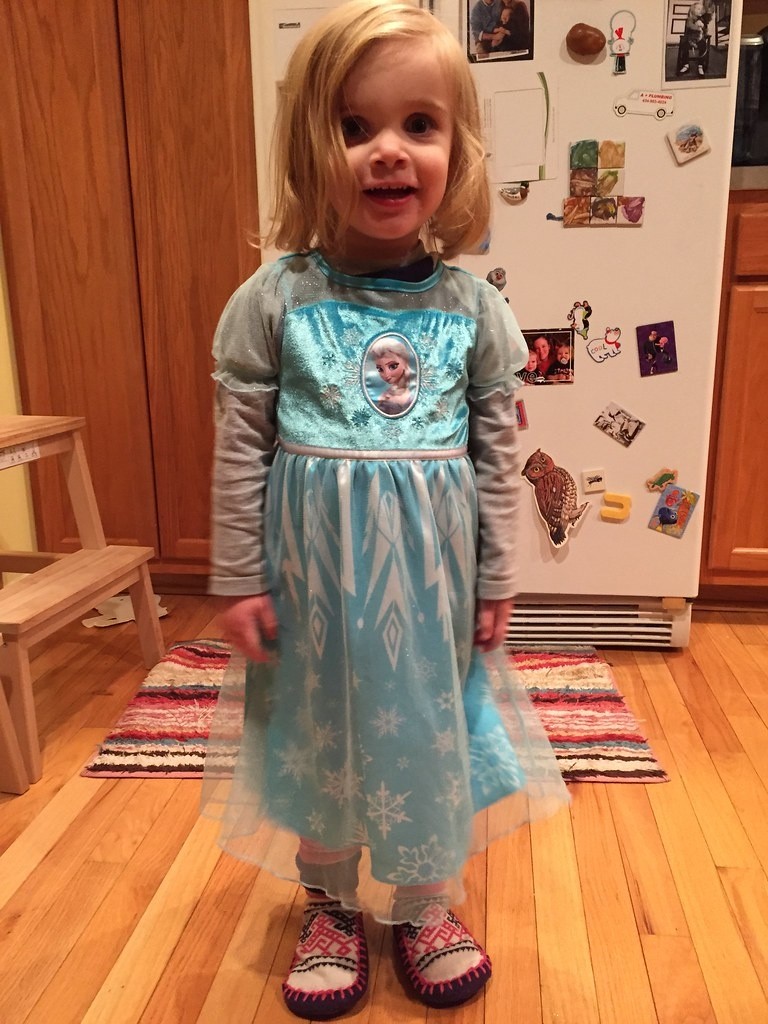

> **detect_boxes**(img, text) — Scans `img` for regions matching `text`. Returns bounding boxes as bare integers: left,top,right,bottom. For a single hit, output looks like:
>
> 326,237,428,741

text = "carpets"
81,638,670,784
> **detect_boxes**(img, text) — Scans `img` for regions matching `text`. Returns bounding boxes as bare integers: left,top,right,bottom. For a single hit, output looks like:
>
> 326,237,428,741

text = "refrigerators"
249,1,744,648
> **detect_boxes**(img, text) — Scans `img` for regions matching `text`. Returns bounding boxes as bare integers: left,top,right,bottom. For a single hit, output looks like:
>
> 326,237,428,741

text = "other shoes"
284,891,367,1021
388,896,492,1006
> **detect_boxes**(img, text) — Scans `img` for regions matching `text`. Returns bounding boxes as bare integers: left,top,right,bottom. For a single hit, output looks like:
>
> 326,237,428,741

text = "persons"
515,328,674,384
471,0,529,53
676,2,710,77
211,2,532,1019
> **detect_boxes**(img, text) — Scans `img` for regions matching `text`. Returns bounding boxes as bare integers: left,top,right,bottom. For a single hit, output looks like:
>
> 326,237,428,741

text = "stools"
677,35,712,69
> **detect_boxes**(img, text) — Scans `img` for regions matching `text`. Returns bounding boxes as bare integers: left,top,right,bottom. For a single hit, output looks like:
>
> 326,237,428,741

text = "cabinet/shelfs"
693,189,768,610
0,0,263,595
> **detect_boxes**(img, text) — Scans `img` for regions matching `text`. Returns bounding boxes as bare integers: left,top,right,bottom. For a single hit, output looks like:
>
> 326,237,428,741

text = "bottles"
736,34,764,128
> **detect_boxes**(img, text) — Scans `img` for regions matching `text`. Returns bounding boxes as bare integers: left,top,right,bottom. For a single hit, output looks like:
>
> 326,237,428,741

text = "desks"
0,413,108,795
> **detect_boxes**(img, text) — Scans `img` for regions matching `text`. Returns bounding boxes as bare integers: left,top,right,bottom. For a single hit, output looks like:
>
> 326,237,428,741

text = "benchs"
0,545,166,783
